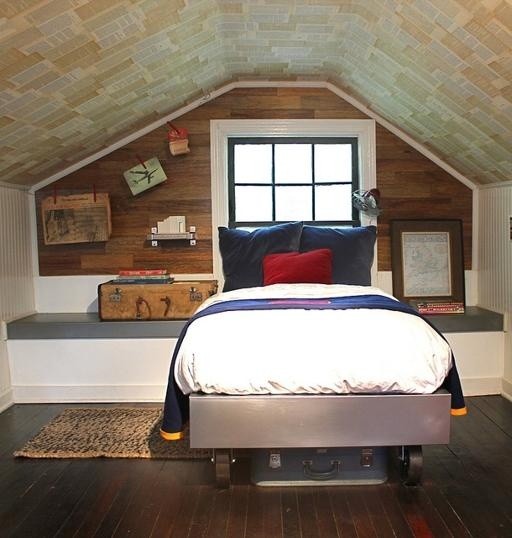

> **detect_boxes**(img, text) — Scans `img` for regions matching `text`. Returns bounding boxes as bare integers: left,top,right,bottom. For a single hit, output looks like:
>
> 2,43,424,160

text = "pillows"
218,221,302,292
261,248,333,286
300,225,376,286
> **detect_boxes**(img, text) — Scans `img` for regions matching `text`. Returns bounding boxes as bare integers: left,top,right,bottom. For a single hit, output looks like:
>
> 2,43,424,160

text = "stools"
417,305,504,397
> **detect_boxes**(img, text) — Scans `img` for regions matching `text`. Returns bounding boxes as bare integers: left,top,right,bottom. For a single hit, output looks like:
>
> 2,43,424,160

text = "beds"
159,220,467,488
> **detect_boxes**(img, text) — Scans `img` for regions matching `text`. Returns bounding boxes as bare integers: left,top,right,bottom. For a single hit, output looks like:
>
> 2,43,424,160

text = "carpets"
12,408,211,460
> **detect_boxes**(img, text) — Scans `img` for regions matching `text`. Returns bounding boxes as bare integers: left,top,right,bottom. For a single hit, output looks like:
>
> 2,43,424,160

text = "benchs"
5,314,192,403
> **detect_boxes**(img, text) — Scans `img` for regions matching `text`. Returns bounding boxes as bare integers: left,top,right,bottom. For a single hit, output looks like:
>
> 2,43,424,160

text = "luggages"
248,448,387,488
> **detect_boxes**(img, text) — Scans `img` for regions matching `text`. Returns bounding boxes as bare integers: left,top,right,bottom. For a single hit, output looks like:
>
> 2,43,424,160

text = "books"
118,268,169,276
116,273,171,280
414,299,465,314
112,278,174,285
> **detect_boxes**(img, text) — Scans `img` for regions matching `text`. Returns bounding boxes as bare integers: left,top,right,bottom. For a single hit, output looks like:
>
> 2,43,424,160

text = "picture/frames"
390,219,465,307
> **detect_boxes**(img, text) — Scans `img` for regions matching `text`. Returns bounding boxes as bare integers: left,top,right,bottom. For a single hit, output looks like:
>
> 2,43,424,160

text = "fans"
352,188,380,217
